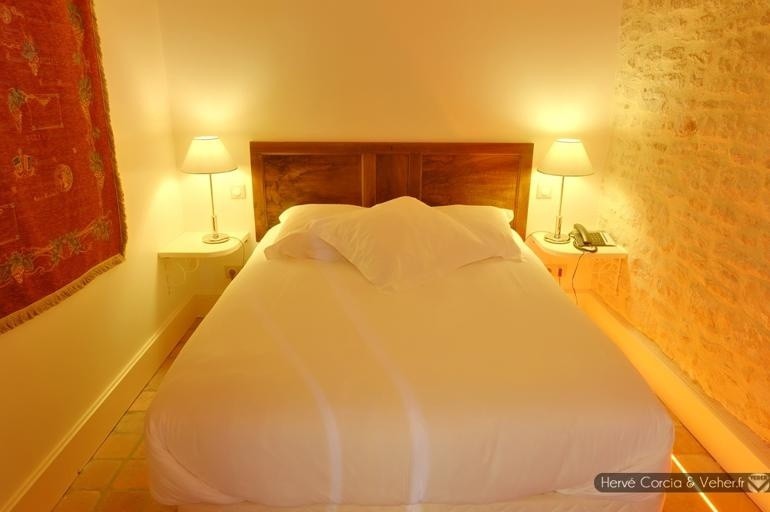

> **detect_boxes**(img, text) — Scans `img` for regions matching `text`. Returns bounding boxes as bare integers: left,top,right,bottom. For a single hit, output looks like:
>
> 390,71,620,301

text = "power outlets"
546,265,567,279
225,267,239,278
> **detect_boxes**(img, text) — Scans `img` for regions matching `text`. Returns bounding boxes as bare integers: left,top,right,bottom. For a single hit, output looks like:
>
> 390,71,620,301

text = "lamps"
537,138,596,245
180,135,238,245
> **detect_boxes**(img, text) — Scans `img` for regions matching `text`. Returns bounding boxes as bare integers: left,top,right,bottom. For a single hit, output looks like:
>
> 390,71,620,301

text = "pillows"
264,204,368,262
430,203,523,260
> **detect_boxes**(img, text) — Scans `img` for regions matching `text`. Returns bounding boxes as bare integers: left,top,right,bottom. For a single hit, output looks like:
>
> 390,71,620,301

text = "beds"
143,224,675,512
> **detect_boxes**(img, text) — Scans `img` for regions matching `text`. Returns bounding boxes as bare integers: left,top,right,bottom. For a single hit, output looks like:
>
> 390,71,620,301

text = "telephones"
573,223,617,247
